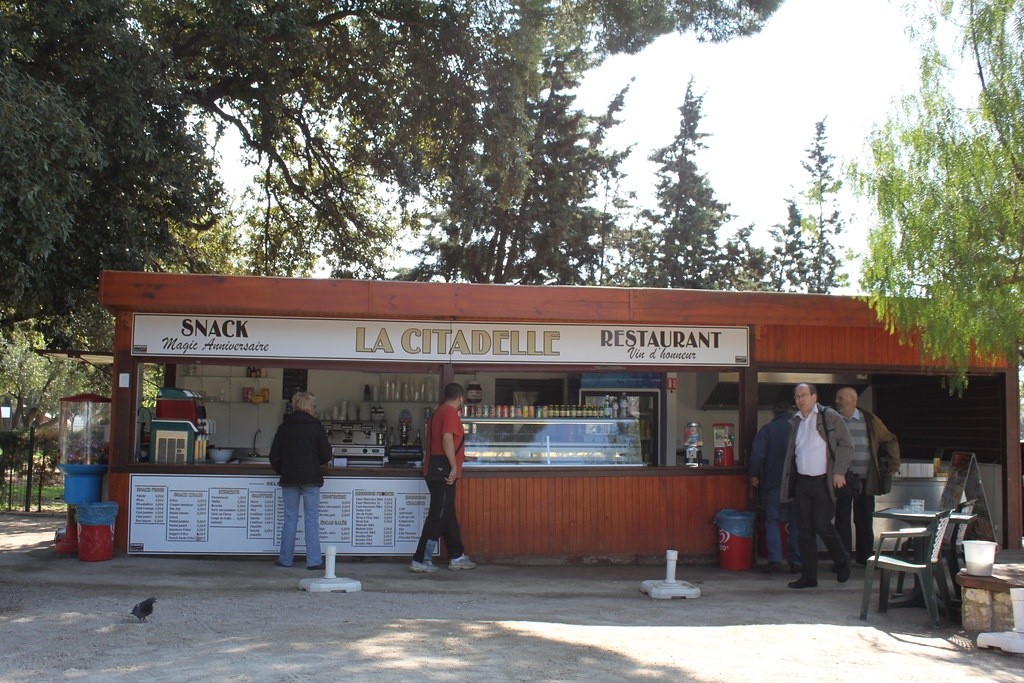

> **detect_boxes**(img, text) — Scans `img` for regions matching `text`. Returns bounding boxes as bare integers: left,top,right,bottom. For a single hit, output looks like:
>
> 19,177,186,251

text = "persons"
750,397,806,574
268,391,333,572
780,383,855,589
831,387,900,571
408,383,477,573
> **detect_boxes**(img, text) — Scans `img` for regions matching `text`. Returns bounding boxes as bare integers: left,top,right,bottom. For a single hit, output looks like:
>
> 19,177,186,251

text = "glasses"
794,392,812,400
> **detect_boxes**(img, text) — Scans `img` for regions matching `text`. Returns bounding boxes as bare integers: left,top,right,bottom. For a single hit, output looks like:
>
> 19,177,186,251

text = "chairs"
860,508,956,629
895,498,978,609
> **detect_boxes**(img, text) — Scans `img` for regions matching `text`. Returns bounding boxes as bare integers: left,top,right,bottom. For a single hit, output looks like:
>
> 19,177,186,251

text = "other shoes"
788,574,817,588
275,559,283,566
791,563,802,574
762,561,782,573
837,569,849,582
308,563,325,570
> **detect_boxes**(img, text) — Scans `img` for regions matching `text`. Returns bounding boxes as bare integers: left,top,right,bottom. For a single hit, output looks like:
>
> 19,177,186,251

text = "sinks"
238,457,270,464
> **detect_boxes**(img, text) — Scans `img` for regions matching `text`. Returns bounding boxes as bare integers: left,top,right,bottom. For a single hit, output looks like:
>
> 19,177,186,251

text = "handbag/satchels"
835,472,863,497
879,468,890,494
427,455,452,482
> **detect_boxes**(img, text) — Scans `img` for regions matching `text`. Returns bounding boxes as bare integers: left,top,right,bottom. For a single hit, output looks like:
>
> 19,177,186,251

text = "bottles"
549,405,604,418
389,426,395,445
425,407,430,423
370,407,384,420
242,386,269,402
604,392,628,418
415,429,422,445
247,366,267,377
220,388,224,402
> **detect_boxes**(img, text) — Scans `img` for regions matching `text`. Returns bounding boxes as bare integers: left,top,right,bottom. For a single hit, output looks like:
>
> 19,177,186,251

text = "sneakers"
410,560,439,573
448,555,476,570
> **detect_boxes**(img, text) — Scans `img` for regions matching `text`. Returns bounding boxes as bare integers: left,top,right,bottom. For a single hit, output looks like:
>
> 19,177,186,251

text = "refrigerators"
579,371,660,466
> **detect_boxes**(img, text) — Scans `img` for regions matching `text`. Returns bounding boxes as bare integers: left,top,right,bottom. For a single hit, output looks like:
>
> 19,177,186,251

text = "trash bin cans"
714,508,763,572
71,502,120,562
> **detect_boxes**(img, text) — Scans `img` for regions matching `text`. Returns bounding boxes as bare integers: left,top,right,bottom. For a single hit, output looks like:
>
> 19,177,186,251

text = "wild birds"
130,597,158,624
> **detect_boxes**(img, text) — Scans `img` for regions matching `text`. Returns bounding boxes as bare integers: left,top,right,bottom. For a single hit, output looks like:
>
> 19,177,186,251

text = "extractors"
696,372,873,410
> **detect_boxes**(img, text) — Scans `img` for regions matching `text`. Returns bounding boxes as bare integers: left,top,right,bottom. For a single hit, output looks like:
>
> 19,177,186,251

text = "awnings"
34,350,116,381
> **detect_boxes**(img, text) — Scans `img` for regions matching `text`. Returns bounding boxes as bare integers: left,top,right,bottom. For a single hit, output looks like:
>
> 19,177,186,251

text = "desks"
871,507,978,617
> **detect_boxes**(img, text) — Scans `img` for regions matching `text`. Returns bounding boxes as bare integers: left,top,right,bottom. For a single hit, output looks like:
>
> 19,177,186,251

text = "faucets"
253,429,262,451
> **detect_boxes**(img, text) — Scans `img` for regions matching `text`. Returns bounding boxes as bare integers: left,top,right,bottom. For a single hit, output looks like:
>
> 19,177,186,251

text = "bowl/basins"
208,397,217,402
208,448,233,463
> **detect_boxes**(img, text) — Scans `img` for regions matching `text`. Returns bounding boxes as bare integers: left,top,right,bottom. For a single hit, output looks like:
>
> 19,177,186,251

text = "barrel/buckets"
466,381,482,402
959,540,1001,575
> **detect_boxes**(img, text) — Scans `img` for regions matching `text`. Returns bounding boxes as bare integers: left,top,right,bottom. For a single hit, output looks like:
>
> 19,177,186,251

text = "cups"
320,400,359,420
903,499,925,512
364,376,439,401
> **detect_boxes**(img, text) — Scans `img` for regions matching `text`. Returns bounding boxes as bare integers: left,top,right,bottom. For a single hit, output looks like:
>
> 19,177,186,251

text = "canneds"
461,404,548,418
